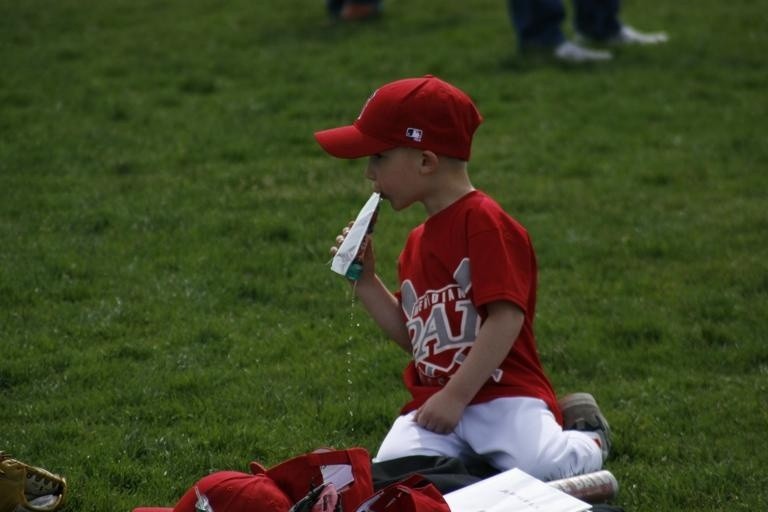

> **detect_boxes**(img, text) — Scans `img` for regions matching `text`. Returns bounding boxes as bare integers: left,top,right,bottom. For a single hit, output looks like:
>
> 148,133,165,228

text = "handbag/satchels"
250,447,451,512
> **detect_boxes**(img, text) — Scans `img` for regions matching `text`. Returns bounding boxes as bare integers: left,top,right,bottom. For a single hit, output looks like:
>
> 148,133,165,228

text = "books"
442,466,593,512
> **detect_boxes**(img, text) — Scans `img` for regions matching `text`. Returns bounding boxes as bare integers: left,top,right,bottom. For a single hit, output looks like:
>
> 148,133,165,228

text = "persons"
312,74,616,483
506,1,670,69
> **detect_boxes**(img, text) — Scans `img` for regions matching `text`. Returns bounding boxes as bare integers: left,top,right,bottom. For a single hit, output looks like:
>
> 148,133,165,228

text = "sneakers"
559,393,616,465
522,25,669,63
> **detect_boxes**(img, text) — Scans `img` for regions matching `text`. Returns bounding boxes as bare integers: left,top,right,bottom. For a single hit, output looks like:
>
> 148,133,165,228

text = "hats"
132,470,293,512
314,74,483,160
